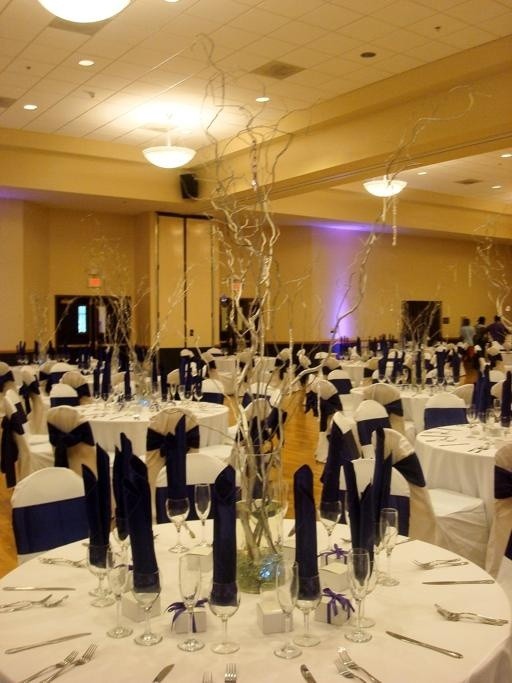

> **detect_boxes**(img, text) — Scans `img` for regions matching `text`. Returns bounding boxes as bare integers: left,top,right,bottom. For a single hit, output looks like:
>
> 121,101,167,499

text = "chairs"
0,342,512,605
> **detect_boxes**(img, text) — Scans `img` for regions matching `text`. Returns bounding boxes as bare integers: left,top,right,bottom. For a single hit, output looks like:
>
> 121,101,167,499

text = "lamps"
143,131,196,169
363,174,407,197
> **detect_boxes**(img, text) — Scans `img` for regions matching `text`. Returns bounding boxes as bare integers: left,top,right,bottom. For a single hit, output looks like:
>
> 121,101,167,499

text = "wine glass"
377,367,461,394
378,508,400,586
207,580,242,655
17,353,71,367
86,544,116,607
85,381,204,411
131,568,163,647
349,560,376,627
318,500,342,555
338,351,350,363
165,497,191,553
274,559,304,659
193,482,212,549
223,347,229,358
466,398,512,442
178,553,205,652
291,572,321,647
343,547,374,643
105,544,133,639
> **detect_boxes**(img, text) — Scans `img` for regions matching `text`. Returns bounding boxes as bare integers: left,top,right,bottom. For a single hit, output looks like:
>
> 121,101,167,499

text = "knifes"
2,584,75,590
5,631,92,654
386,629,463,658
422,580,496,584
300,664,318,682
151,662,175,682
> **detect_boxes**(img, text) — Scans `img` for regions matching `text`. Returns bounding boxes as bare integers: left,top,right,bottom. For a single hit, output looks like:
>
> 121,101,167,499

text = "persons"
460,315,508,370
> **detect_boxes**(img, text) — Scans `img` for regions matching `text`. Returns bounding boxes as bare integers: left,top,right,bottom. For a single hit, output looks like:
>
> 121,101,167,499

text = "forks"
39,556,86,569
223,662,237,682
333,657,367,682
0,593,72,613
38,640,97,682
412,556,469,569
18,648,80,682
200,671,213,682
434,600,511,626
338,647,382,682
